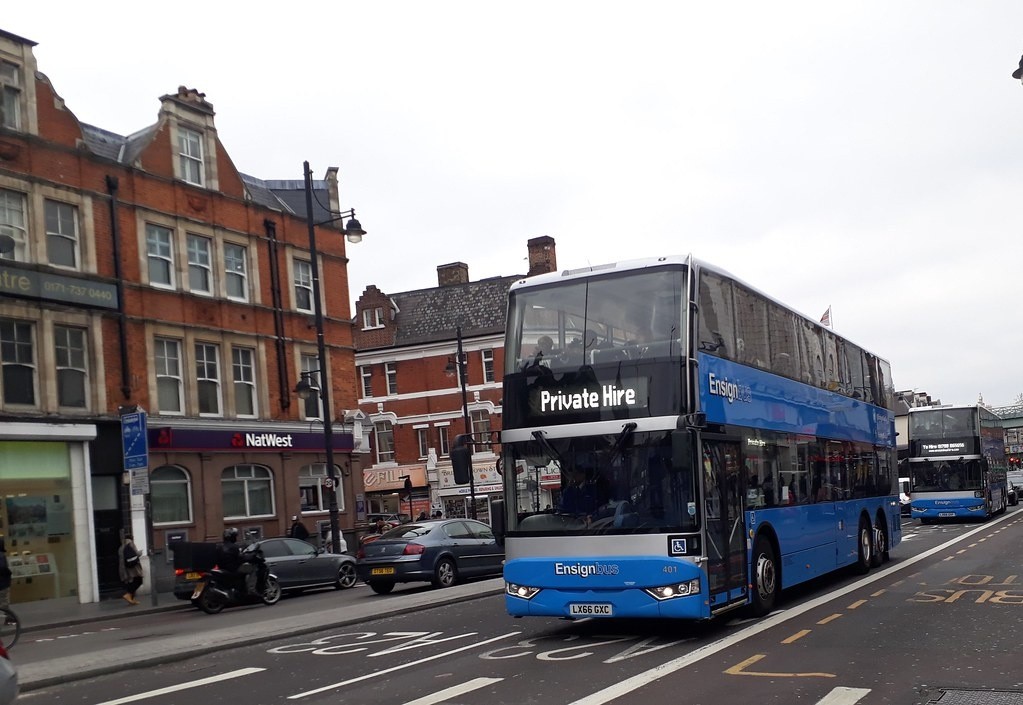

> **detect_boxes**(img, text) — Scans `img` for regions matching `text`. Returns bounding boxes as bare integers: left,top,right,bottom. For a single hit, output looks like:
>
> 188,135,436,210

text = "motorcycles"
191,543,281,614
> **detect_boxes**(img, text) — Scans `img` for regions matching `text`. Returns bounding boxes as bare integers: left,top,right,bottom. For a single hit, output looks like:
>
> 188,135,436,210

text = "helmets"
222,527,239,543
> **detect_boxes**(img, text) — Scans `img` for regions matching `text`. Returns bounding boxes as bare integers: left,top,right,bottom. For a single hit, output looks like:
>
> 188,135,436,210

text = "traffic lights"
1010,457,1014,462
1015,458,1020,463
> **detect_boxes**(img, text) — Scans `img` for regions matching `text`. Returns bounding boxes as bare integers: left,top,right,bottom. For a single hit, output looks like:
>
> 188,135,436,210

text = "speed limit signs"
324,477,333,488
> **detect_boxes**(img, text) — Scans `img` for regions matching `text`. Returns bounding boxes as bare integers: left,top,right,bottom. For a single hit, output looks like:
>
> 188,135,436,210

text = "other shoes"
123,593,141,605
4,616,17,625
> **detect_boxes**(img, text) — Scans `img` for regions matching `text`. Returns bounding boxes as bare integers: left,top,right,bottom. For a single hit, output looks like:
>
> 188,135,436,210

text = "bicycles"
0,606,21,651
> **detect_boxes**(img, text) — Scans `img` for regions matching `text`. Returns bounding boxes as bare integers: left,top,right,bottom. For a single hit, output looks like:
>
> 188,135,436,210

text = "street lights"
292,161,367,554
443,326,477,520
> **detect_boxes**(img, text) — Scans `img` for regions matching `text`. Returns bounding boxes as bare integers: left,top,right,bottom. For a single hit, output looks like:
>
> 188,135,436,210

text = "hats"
291,515,299,521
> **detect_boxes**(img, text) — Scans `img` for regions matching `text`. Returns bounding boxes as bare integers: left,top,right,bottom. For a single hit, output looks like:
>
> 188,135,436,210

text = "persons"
326,524,347,554
749,473,821,504
402,514,408,523
416,511,442,521
582,330,605,349
534,336,557,356
0,540,17,626
557,468,593,525
290,516,309,539
119,535,143,604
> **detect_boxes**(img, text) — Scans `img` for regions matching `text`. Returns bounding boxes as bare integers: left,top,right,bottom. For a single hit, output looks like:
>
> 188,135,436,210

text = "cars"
173,536,358,607
1007,479,1019,505
354,518,505,594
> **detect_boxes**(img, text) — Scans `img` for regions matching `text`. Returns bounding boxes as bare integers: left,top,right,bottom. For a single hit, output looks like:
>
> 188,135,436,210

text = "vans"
899,477,915,513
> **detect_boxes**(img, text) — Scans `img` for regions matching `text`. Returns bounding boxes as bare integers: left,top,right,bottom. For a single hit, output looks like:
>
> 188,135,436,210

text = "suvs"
366,513,414,534
1007,475,1023,497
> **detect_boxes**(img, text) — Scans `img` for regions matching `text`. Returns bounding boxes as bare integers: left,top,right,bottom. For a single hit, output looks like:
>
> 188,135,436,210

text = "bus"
449,252,902,619
902,403,1007,525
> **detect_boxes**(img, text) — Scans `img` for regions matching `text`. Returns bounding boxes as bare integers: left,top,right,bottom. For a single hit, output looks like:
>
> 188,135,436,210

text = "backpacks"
124,544,139,569
